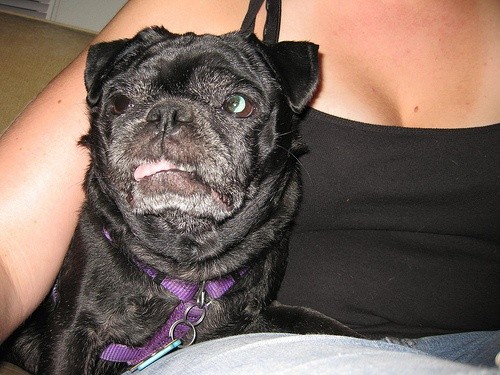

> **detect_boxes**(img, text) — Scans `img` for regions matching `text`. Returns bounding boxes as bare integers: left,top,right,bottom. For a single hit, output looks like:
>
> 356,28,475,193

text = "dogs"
0,26,373,375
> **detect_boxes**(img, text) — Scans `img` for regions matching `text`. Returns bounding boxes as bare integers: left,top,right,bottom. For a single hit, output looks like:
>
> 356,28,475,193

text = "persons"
1,0,500,374
